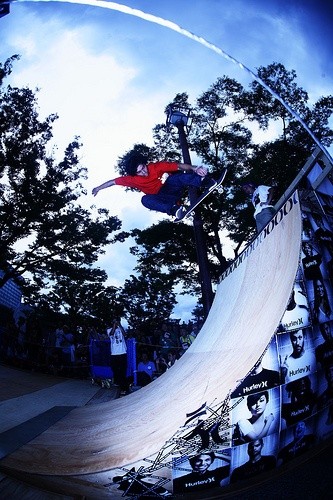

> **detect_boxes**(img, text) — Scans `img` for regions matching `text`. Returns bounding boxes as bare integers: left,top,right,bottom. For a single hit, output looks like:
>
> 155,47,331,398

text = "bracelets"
195,166,203,172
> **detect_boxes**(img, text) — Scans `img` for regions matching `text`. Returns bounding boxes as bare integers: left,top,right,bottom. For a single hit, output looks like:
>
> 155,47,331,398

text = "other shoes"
170,204,189,220
121,390,126,394
200,167,225,193
113,383,119,386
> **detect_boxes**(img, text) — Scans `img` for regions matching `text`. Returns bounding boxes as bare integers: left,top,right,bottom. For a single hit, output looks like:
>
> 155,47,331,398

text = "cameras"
114,321,118,325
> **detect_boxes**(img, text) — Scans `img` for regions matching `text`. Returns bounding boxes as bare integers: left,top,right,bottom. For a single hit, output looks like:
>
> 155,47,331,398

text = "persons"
281,328,317,384
150,324,180,362
280,421,317,460
173,451,231,500
231,439,277,491
319,369,333,408
280,241,333,331
92,156,208,219
107,316,128,398
242,183,275,232
282,376,316,426
234,391,276,445
302,192,333,257
0,318,32,370
56,325,107,362
315,322,333,368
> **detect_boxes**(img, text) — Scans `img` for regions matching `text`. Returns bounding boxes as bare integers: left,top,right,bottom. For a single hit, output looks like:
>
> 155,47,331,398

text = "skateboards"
171,167,229,225
